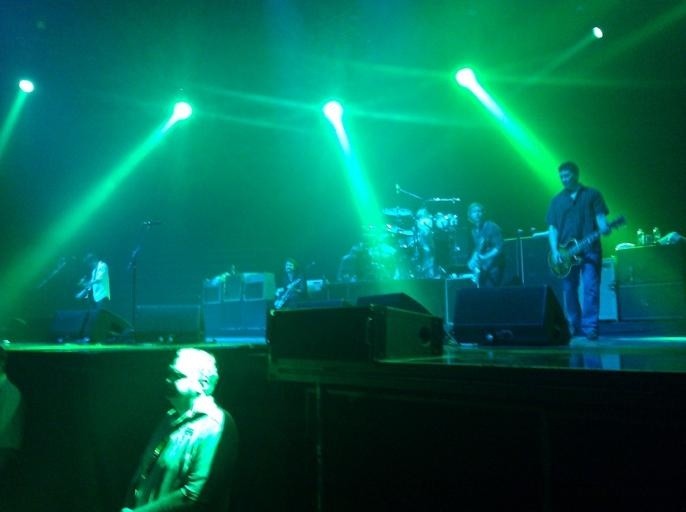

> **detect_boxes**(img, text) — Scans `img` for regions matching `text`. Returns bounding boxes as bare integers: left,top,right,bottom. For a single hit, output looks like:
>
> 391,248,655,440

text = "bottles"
652,226,660,244
636,228,644,245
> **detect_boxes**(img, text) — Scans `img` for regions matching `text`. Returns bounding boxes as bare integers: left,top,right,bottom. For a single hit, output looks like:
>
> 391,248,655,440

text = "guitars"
547,215,625,279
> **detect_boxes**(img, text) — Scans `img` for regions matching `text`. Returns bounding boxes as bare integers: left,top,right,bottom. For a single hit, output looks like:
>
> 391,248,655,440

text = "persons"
1,345,25,485
120,347,242,512
275,258,307,300
467,203,507,287
78,253,111,342
547,162,610,342
336,248,368,281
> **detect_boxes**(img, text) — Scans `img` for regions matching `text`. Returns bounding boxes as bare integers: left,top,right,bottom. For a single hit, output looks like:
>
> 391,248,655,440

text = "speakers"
48,309,133,342
616,245,686,321
454,285,571,346
265,306,445,359
134,305,204,342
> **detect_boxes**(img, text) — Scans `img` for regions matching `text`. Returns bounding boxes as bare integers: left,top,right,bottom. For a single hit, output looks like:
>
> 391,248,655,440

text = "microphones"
145,222,160,225
450,198,460,202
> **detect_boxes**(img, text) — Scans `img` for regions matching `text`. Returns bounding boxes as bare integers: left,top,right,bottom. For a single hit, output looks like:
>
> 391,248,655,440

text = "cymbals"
387,225,414,236
383,208,411,216
